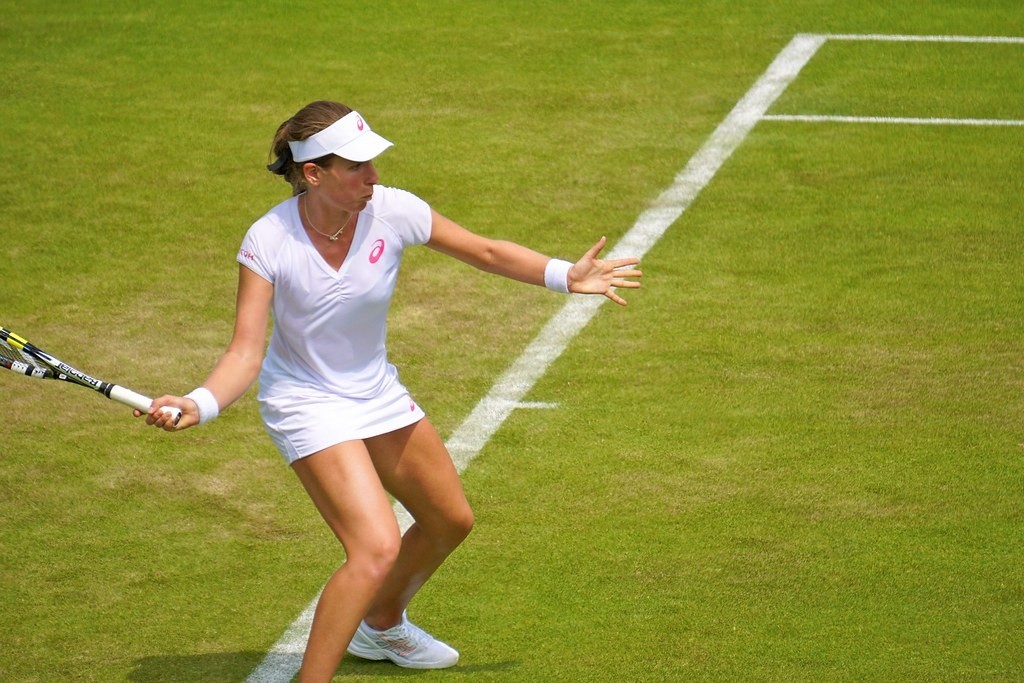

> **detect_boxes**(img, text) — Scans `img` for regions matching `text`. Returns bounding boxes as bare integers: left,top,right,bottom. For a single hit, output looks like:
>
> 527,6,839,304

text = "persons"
134,100,644,683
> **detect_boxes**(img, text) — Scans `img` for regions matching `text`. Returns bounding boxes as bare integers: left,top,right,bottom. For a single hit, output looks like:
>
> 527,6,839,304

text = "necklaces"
304,194,353,241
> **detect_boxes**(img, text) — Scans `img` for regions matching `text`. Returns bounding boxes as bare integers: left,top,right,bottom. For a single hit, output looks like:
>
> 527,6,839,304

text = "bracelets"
544,258,575,294
183,387,219,427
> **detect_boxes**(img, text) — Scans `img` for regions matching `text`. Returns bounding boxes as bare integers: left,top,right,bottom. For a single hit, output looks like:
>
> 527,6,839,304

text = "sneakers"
346,618,460,671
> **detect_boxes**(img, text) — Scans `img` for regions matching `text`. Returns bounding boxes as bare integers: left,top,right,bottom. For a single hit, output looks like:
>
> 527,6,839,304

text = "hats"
265,110,398,174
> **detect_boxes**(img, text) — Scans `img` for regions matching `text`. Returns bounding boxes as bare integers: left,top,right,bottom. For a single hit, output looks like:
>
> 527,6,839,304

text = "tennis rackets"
0,323,181,429
